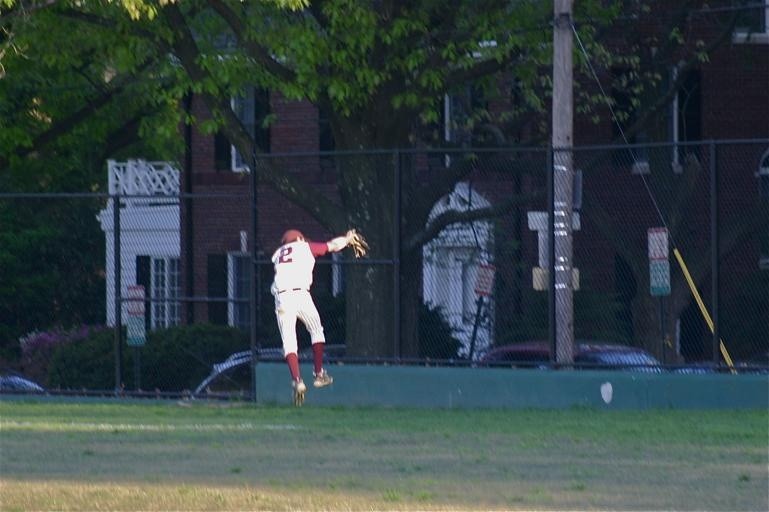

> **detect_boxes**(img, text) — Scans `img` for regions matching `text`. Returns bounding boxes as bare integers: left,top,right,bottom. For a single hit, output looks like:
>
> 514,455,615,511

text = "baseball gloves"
348,228,370,258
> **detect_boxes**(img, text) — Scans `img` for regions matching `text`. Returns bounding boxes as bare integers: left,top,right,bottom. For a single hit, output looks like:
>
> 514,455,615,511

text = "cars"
479,345,769,375
0,373,49,394
193,348,353,403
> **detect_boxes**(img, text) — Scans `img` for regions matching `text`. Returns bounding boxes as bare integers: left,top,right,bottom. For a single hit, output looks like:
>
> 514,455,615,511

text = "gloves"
348,233,371,259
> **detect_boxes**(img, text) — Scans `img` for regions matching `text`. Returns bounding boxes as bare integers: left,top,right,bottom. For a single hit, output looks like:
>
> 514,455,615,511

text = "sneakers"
313,367,333,387
294,378,306,407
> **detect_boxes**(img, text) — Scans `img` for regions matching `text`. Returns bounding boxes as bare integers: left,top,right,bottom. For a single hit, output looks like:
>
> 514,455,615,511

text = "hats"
282,229,303,243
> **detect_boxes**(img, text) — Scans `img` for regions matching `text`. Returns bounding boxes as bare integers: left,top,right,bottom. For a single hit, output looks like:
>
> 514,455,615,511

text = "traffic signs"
649,260,671,296
125,285,147,314
126,314,148,349
473,263,497,297
648,229,670,259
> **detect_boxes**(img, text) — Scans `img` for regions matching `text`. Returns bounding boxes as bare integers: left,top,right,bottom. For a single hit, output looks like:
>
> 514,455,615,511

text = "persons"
271,228,361,407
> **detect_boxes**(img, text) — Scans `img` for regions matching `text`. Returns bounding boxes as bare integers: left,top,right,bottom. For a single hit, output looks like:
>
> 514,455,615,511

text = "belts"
277,288,310,294
